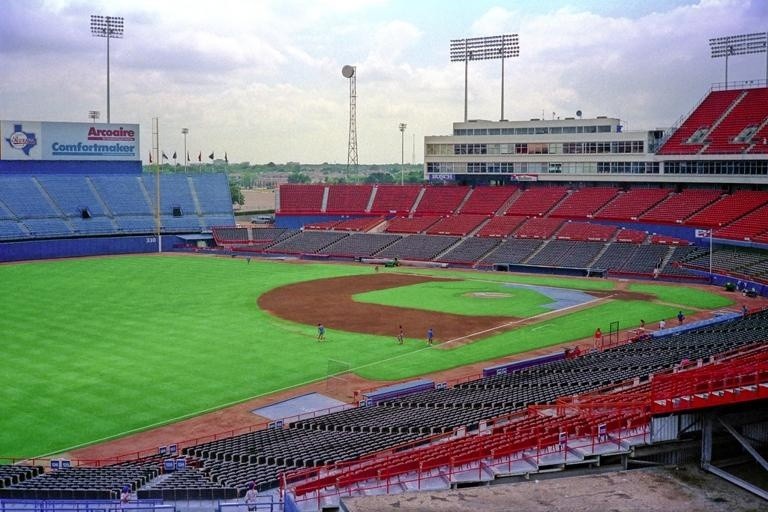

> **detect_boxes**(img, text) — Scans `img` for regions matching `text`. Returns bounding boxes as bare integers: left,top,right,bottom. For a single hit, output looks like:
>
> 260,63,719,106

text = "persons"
398,326,404,344
565,311,684,360
318,324,324,340
428,329,434,345
120,487,130,505
244,482,258,511
741,305,748,320
393,257,399,266
247,256,250,264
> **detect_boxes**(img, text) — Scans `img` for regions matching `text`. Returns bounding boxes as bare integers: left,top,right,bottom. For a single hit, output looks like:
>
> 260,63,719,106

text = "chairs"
0,311,768,508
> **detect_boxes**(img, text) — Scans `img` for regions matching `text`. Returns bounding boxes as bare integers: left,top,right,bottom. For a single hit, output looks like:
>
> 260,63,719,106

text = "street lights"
89,110,100,123
182,127,189,172
399,121,407,186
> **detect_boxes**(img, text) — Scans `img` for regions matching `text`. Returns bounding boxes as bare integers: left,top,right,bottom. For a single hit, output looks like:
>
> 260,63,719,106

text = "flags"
149,151,227,163
